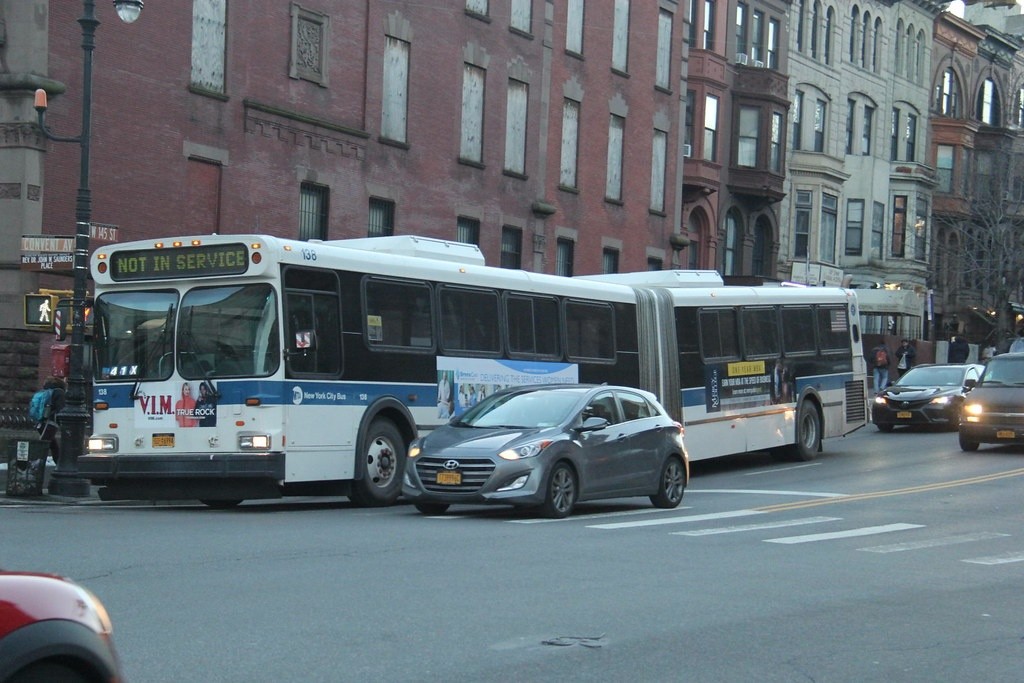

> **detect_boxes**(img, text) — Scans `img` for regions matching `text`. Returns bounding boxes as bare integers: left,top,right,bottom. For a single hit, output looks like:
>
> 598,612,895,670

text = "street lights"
47,0,146,498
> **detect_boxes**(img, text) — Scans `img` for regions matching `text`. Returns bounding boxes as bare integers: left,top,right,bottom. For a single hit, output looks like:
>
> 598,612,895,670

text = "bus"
52,231,874,504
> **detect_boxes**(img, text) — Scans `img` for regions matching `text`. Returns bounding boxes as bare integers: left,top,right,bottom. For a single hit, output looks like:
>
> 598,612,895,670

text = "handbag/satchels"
897,354,907,369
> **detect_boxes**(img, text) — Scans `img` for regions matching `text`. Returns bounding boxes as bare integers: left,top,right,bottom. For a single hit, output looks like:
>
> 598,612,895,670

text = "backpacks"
877,346,889,366
31,388,65,422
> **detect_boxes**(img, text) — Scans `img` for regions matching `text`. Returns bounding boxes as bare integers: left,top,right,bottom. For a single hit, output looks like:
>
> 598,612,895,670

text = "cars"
0,567,124,683
403,383,690,512
958,352,1024,454
870,360,986,433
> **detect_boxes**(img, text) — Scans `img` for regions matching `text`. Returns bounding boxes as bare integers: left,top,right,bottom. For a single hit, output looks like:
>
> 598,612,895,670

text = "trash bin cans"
4,437,52,497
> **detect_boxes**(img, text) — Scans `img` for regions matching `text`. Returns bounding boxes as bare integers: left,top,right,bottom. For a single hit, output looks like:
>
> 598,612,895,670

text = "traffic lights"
23,295,56,328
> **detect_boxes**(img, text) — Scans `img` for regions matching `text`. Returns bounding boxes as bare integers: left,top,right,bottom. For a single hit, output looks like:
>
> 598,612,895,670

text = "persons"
897,338,916,377
869,341,892,396
980,341,997,360
30,376,67,470
781,367,793,403
192,382,217,428
476,385,486,403
495,385,501,393
175,383,198,428
949,333,971,364
436,371,451,405
468,385,477,407
769,360,782,404
458,385,466,408
